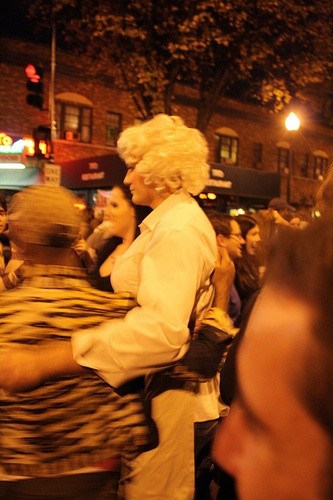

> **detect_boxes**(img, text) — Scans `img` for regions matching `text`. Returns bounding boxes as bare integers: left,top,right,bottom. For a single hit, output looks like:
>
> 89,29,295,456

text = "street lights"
284,112,301,204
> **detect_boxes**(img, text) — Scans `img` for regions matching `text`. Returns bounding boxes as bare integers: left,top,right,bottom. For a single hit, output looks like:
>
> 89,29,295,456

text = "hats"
4,182,85,247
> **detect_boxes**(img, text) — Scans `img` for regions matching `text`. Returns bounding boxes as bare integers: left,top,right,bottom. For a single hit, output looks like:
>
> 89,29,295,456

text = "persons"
283,212,302,227
218,161,333,405
212,211,333,500
237,215,262,308
0,113,221,500
0,183,240,500
0,196,12,270
211,211,246,313
88,184,154,294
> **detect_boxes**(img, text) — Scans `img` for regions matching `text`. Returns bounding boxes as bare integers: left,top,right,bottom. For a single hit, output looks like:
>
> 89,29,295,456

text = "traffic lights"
23,62,49,110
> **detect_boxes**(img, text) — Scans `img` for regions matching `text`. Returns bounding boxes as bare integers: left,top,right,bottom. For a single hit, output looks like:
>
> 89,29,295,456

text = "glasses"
226,233,243,239
